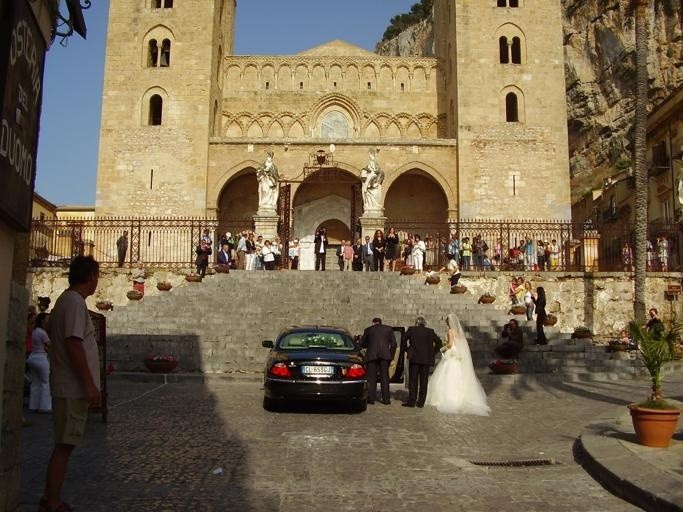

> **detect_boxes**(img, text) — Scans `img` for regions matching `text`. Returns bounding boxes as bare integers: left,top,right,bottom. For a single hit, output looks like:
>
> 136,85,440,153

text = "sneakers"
38,496,75,512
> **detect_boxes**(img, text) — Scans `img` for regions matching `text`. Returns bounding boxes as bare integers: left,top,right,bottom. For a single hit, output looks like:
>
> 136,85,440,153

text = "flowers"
609,337,631,345
301,334,336,346
489,359,518,369
143,353,177,362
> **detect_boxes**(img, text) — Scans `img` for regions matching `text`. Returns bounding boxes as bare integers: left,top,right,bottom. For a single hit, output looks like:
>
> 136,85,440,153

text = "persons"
425,313,492,417
618,330,630,345
646,308,665,338
357,318,397,405
440,254,461,286
314,227,328,271
425,266,437,285
193,227,302,278
495,319,523,359
361,148,383,193
622,233,669,272
39,254,103,512
510,277,548,346
24,305,53,414
117,230,128,267
131,261,145,295
336,227,560,271
256,150,280,189
402,318,442,408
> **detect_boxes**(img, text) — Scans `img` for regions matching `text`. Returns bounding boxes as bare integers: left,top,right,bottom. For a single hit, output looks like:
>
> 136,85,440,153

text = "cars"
260,324,408,415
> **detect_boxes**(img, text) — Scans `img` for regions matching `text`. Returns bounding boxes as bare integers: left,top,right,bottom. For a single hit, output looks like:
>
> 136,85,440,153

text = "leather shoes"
368,400,424,409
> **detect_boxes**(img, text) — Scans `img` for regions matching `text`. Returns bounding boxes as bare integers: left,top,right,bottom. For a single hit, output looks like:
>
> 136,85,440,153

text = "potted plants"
624,288,683,449
401,266,590,337
96,263,229,311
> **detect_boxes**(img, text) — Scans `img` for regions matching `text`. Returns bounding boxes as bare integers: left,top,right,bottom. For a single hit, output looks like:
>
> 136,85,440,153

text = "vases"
491,366,515,374
144,362,175,372
612,344,629,350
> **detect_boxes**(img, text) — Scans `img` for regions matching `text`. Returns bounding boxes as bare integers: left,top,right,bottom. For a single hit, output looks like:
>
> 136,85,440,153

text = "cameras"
505,323,511,328
